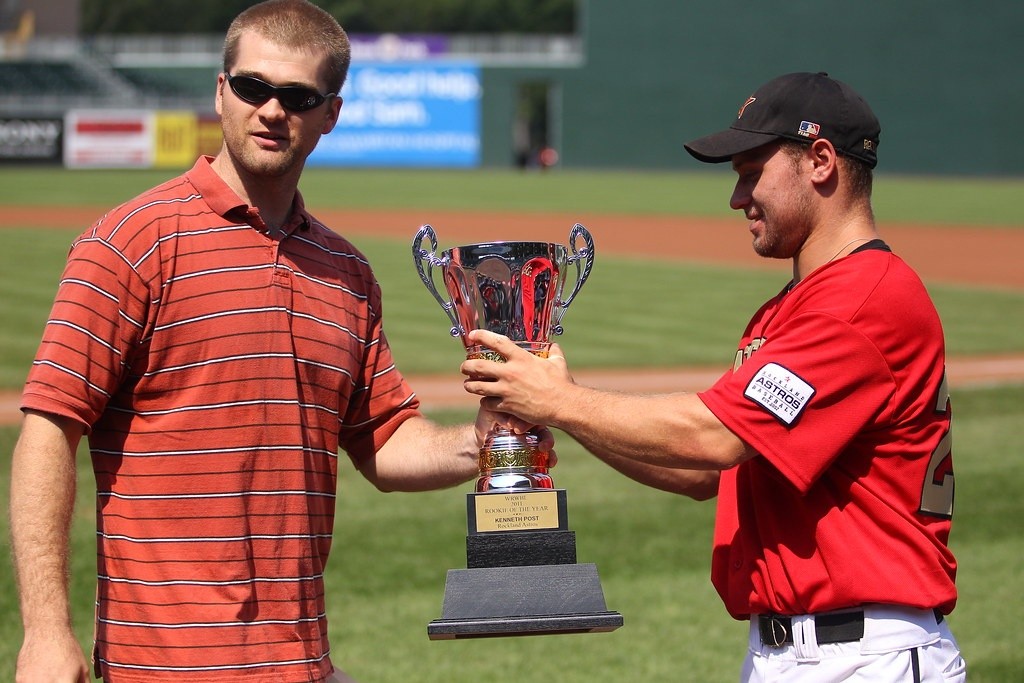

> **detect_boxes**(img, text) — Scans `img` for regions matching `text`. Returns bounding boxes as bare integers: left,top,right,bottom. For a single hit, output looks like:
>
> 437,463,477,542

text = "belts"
759,608,944,648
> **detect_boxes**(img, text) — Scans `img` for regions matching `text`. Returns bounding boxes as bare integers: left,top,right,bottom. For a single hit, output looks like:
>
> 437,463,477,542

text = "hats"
683,71,881,167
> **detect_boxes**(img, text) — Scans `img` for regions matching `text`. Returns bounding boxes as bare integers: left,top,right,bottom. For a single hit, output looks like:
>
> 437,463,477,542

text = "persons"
460,74,969,683
10,0,558,683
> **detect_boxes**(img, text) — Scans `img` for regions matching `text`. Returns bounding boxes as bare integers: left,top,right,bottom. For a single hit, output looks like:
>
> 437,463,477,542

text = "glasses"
224,71,337,114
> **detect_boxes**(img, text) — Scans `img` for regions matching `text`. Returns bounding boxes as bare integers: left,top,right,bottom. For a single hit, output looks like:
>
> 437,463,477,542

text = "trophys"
411,224,626,641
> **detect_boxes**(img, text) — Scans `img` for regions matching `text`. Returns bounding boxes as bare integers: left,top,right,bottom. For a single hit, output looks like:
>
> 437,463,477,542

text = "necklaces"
788,238,868,293
